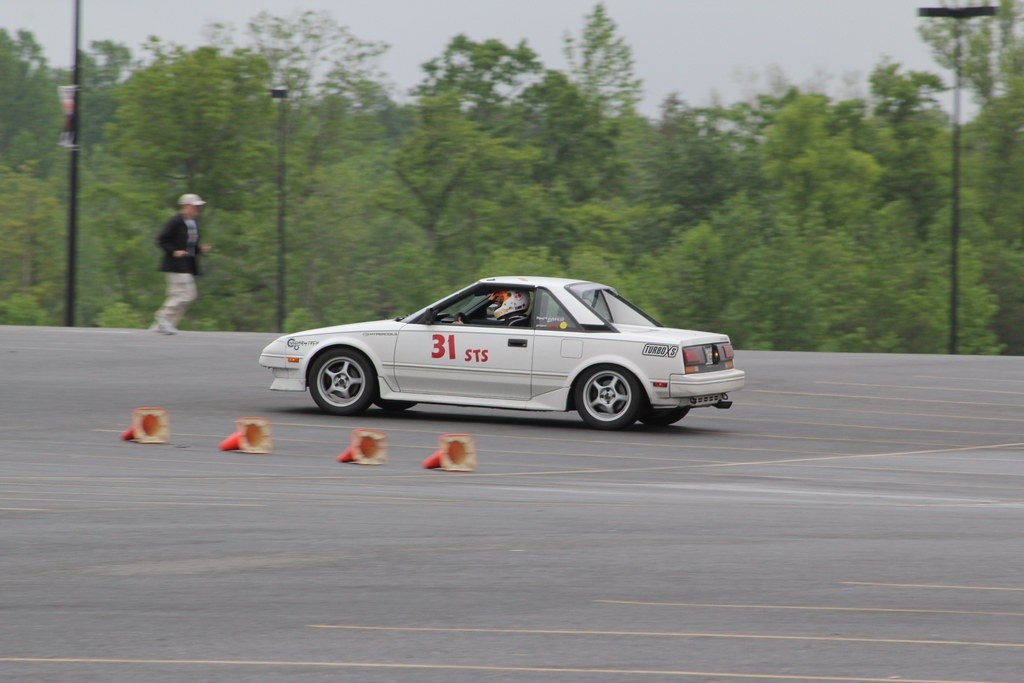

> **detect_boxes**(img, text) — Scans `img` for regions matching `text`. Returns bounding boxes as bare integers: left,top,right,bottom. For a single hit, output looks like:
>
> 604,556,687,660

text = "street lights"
270,83,291,333
912,6,1001,355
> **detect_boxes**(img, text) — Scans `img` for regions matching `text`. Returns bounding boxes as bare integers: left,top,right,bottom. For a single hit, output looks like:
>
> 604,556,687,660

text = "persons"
452,288,531,329
149,193,213,335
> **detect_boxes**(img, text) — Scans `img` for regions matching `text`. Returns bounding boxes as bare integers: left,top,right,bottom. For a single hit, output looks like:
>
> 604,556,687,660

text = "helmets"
487,289,530,320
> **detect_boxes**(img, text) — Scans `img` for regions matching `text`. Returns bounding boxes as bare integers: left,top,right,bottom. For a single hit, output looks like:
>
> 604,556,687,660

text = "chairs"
528,293,549,324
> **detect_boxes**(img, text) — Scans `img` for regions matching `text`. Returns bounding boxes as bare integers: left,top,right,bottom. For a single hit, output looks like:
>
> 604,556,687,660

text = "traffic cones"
337,428,390,466
218,416,276,456
118,404,173,447
419,434,480,473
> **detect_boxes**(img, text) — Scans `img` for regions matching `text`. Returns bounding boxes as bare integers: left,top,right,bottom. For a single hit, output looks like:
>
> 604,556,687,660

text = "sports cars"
260,274,749,434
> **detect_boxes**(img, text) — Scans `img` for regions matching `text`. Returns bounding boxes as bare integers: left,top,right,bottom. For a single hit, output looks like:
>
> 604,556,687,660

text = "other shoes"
146,316,178,335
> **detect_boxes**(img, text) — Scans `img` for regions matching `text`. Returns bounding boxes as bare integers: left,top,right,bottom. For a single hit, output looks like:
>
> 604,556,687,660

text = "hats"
177,192,206,206
